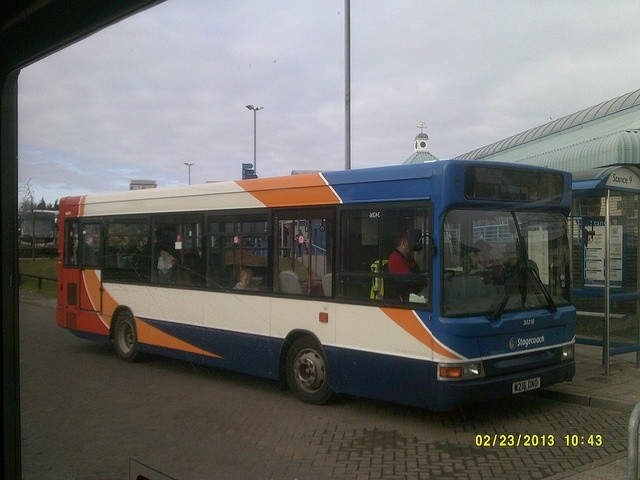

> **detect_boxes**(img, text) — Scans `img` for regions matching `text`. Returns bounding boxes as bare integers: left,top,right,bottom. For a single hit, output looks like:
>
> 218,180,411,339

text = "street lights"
246,104,265,175
183,162,196,186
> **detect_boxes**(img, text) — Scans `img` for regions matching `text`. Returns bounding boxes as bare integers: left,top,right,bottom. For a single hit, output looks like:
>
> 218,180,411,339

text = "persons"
232,268,260,291
549,256,557,297
560,255,570,301
388,233,419,276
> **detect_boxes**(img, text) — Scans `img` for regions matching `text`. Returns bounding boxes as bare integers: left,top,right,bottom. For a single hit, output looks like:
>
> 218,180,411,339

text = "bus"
17,208,59,257
56,159,577,414
